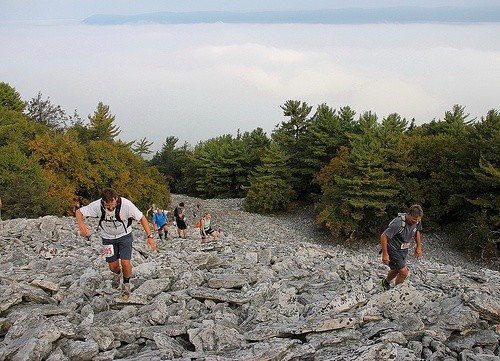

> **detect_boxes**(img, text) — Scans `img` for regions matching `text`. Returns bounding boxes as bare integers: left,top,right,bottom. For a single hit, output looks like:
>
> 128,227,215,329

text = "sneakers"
121,283,131,301
380,278,390,291
111,273,120,290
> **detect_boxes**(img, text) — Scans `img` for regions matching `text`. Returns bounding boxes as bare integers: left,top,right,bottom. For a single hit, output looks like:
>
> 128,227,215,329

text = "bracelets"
147,234,153,238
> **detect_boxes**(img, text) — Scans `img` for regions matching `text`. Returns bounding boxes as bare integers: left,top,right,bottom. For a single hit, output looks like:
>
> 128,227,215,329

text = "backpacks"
173,207,178,218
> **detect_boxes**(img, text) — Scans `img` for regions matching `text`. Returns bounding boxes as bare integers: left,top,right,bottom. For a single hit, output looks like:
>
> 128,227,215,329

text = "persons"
192,203,200,219
174,202,187,239
380,204,423,290
200,211,217,244
146,204,169,241
76,188,157,296
